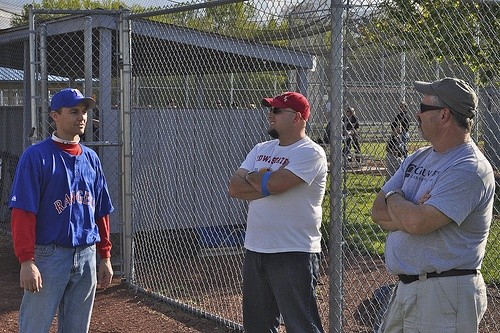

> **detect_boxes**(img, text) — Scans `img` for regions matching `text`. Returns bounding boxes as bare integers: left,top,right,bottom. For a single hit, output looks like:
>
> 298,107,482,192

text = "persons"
384,102,411,185
318,107,363,162
371,78,496,333
228,91,328,333
10,88,114,333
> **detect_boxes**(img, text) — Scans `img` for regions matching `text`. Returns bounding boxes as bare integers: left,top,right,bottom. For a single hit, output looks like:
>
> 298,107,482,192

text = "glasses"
270,108,298,115
419,102,445,112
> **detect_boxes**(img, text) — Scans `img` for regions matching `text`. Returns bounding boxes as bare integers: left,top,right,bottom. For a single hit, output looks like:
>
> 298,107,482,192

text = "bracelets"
246,170,255,175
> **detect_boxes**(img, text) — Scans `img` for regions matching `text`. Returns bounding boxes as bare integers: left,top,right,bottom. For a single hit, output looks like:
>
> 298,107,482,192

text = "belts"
397,268,477,285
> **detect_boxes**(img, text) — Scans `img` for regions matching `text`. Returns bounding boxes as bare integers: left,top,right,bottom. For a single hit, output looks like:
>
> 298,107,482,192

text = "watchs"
385,190,400,204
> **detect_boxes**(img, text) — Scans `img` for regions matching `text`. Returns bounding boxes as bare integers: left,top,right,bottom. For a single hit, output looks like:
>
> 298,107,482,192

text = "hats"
413,77,478,119
261,92,309,121
50,88,97,112
